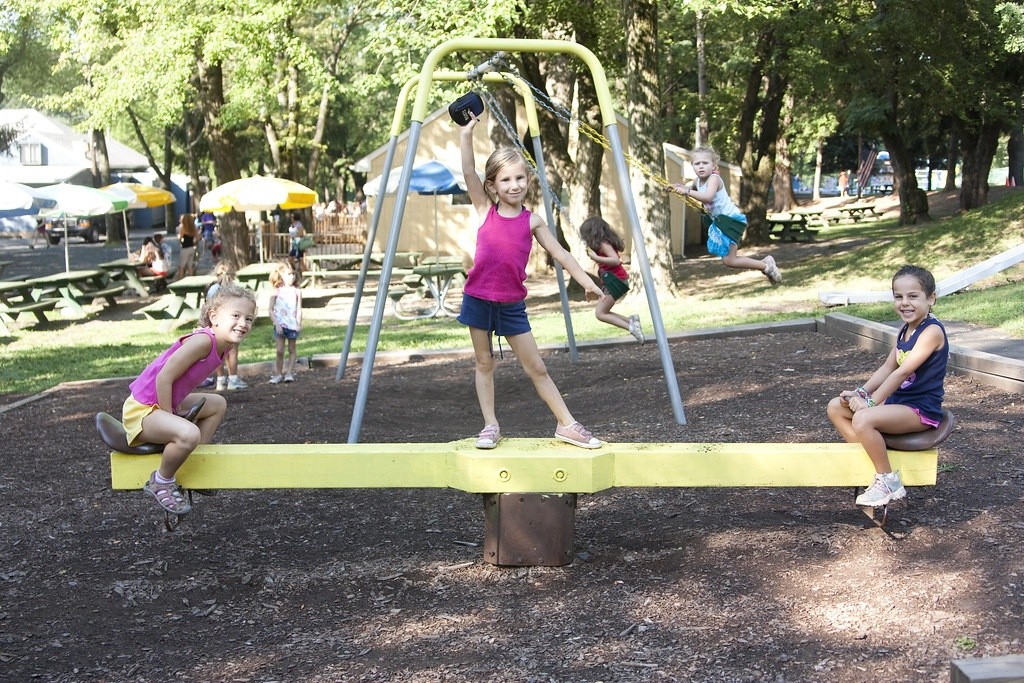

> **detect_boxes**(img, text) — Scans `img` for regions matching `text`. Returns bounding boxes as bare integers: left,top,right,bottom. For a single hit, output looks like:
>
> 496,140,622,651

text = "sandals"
143,470,192,514
193,489,218,496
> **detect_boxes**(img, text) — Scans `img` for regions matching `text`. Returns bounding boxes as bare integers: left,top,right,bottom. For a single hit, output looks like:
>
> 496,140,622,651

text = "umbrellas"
199,174,318,264
98,182,176,258
363,160,484,266
0,183,62,218
34,183,129,272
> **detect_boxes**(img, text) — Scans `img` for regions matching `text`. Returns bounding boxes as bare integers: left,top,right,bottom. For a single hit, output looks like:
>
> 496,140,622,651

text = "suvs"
45,212,131,243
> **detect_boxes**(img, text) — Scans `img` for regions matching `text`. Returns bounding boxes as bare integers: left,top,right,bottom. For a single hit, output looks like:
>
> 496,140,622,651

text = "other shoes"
285,375,294,382
269,375,282,384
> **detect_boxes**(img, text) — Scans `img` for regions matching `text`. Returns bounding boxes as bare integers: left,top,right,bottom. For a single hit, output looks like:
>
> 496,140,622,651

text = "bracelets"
856,386,877,407
686,189,690,196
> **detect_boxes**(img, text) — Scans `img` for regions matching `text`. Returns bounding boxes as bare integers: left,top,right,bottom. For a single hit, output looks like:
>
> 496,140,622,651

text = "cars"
793,186,870,196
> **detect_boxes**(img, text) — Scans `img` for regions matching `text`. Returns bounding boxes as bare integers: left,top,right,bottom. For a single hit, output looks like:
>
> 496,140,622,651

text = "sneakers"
476,425,502,449
217,377,228,391
196,379,216,388
228,377,249,390
555,422,602,449
761,255,782,285
855,469,906,506
630,313,645,346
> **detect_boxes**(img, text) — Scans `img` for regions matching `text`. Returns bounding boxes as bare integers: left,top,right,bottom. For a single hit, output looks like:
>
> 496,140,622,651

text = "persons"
457,112,606,449
27,217,51,248
827,265,950,507
839,169,854,198
286,213,308,278
131,212,224,278
194,260,249,391
122,281,258,514
663,146,783,288
268,264,303,382
579,217,645,346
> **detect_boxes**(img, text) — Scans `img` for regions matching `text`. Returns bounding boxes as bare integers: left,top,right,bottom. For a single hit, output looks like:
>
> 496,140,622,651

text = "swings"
487,50,748,247
467,65,631,303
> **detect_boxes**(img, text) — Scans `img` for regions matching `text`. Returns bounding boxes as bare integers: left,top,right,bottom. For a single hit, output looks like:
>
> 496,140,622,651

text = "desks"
412,265,465,315
840,206,875,213
0,282,49,323
99,258,147,269
787,212,822,221
768,220,807,235
167,276,218,308
422,256,465,266
237,262,283,287
25,270,104,292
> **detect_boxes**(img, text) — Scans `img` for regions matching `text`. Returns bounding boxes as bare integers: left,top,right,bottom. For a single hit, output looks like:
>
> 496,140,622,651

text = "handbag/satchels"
450,92,484,126
298,236,315,251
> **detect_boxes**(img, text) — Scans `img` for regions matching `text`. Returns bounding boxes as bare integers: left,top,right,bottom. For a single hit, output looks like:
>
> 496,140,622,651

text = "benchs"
827,210,883,223
769,223,822,241
0,274,472,334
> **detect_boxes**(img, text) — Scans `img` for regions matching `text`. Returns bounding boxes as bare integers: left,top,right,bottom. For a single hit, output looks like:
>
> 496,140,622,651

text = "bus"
870,150,895,193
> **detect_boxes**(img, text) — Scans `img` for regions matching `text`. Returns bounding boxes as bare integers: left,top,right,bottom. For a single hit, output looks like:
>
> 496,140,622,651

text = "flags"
857,145,878,188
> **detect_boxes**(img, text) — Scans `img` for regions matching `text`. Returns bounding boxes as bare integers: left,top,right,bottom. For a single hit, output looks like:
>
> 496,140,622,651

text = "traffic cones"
1006,176,1016,186
